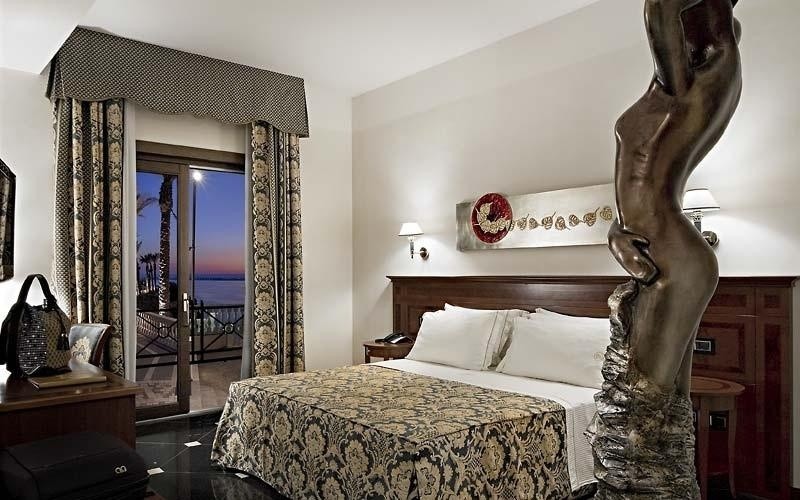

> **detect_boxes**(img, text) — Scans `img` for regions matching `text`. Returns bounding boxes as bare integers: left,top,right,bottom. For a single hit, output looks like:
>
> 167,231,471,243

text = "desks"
689,376,747,500
363,340,413,364
0,365,148,498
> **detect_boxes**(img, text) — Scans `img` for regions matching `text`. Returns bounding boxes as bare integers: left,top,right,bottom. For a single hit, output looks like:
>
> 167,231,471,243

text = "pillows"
404,301,612,391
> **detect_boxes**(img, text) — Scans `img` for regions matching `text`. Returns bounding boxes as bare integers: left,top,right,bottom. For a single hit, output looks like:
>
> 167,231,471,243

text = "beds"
230,356,606,500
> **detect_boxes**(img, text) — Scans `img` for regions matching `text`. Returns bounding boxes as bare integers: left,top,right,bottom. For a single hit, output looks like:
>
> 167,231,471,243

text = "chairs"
69,323,115,367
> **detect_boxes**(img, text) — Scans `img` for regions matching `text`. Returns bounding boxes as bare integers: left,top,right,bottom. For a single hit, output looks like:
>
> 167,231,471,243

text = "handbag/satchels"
1,273,74,379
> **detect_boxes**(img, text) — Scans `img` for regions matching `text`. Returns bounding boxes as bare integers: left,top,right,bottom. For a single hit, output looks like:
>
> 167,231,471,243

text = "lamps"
681,188,722,248
398,223,429,261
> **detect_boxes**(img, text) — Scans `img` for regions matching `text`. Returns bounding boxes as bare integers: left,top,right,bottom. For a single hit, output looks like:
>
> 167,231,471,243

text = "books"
28,370,108,390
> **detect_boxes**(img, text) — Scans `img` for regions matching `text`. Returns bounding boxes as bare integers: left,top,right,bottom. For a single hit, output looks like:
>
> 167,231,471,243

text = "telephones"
384,333,409,344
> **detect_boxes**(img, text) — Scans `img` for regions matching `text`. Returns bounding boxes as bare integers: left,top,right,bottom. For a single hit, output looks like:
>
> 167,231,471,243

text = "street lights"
190,167,203,321
